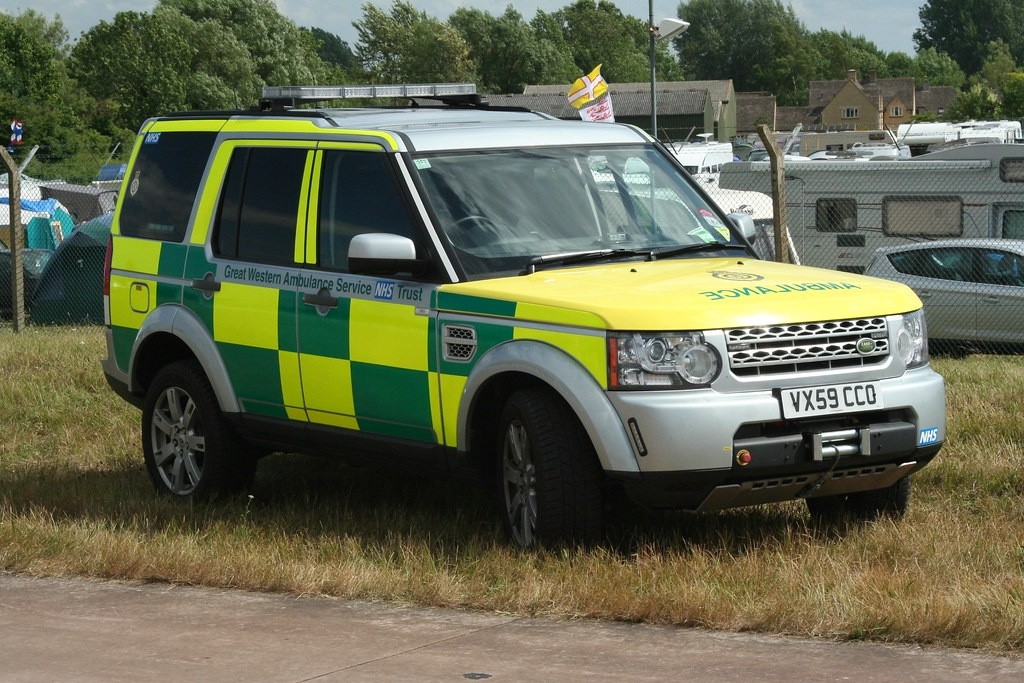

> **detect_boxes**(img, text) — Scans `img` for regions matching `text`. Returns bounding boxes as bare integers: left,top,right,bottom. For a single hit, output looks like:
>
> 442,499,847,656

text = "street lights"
646,15,690,142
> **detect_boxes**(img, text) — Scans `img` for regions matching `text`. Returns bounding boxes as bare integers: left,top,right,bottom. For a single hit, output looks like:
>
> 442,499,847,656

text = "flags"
578,95,615,122
568,64,608,108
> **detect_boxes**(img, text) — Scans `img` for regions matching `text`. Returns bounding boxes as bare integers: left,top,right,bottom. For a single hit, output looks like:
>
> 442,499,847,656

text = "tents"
0,162,125,325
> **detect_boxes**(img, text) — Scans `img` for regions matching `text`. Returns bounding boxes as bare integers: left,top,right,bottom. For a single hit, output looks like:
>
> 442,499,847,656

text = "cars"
863,237,1024,347
0,247,57,321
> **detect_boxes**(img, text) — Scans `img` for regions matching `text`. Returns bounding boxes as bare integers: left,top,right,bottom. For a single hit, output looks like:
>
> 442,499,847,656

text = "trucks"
591,111,1023,277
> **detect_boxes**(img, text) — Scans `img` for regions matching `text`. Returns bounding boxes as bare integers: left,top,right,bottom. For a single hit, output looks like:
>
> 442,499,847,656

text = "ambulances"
97,83,949,558
